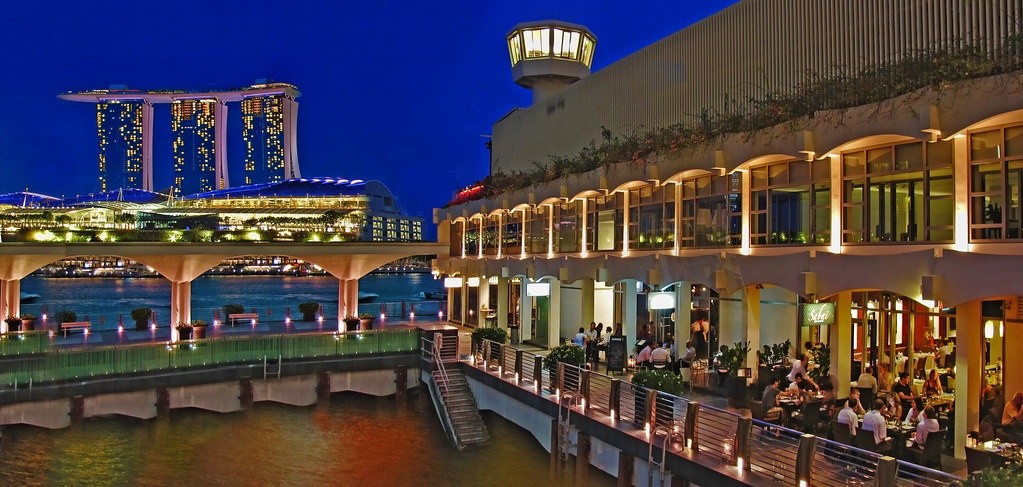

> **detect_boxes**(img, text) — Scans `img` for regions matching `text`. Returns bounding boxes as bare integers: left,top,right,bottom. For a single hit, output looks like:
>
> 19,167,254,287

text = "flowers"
541,338,588,371
631,368,684,397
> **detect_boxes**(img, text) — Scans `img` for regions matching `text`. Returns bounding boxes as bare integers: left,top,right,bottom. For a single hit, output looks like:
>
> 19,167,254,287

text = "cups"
992,437,1006,456
785,387,812,399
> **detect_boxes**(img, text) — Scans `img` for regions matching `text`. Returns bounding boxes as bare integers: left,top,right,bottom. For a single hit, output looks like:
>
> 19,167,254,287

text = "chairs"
565,338,1023,487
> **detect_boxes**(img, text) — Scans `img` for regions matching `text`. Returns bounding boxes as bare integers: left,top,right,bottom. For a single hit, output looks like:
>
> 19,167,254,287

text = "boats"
358,291,380,303
421,291,448,300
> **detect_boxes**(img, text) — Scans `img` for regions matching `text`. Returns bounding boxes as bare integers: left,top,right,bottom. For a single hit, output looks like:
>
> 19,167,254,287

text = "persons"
572,341,1023,475
576,316,716,373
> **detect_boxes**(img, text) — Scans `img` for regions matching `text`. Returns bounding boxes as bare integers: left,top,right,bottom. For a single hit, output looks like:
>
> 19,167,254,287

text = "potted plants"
5,303,378,350
471,326,509,360
807,342,833,391
717,341,752,409
757,339,792,389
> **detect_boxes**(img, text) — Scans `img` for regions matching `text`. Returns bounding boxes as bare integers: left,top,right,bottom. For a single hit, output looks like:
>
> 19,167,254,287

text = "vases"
634,387,675,431
550,366,588,396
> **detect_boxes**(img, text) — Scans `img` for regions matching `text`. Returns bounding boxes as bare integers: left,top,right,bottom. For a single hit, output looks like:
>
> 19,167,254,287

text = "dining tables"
830,414,917,460
914,353,939,369
894,377,926,396
907,394,955,429
778,399,830,427
925,368,949,381
964,442,1023,474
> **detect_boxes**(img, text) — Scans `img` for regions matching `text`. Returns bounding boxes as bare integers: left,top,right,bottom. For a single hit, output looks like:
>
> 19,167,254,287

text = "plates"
886,421,918,429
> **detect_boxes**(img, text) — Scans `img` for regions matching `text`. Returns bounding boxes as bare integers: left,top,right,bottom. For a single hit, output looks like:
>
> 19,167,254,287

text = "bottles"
919,387,955,404
966,431,984,449
897,421,902,432
712,355,721,364
912,418,917,426
886,415,896,424
589,330,595,339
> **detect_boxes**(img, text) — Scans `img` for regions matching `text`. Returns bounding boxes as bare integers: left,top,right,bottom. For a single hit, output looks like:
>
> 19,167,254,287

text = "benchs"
229,313,257,327
61,322,92,339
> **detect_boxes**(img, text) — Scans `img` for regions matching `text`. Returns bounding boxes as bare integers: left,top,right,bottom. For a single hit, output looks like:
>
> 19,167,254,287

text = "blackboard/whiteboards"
607,335,626,371
